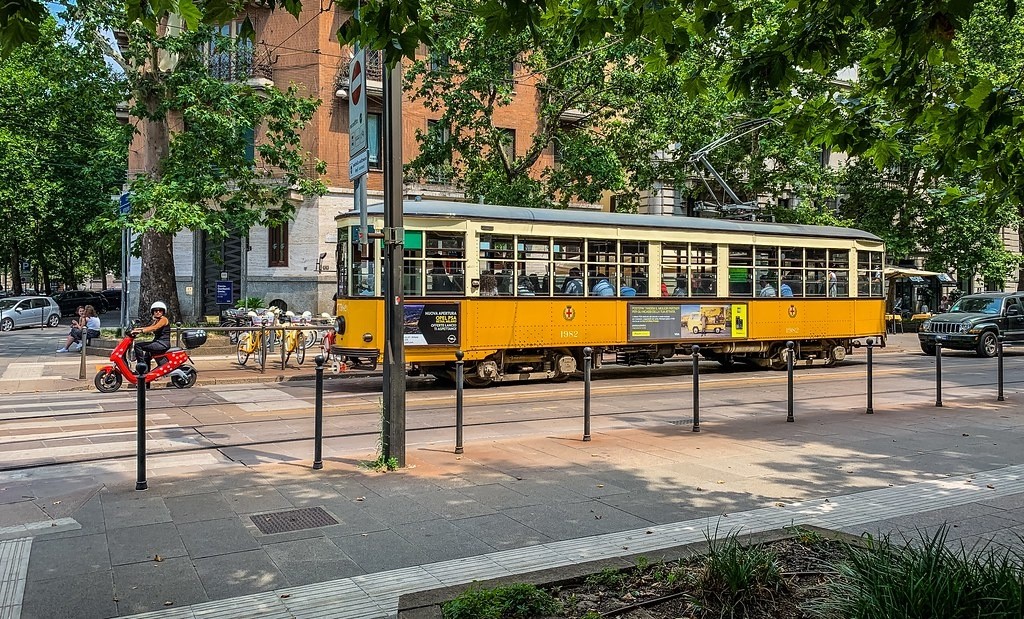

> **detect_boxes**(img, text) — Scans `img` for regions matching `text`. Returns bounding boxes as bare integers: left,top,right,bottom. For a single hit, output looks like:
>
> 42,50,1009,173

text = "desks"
911,314,931,332
885,315,901,333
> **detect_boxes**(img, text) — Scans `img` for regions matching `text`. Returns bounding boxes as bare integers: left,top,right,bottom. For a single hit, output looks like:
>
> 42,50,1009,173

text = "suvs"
917,290,1024,358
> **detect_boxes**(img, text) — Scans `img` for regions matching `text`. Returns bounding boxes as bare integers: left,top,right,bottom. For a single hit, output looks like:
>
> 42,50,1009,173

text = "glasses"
155,311,162,313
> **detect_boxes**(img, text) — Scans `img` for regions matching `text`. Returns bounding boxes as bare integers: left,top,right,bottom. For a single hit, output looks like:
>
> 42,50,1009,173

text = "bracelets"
141,329,146,334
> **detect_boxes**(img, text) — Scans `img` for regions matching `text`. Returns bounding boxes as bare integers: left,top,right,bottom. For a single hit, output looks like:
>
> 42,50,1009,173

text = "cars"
0,287,127,317
0,297,62,331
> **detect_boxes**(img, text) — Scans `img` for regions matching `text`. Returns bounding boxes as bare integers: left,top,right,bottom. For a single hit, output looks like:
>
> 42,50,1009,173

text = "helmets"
150,301,167,313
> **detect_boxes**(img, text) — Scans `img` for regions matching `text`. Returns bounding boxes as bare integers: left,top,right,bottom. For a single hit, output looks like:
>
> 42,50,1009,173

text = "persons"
939,296,948,313
432,253,452,290
780,262,883,298
758,275,776,297
56,305,101,354
671,275,688,296
130,301,170,389
591,273,648,296
480,267,585,296
814,262,838,297
661,283,669,296
695,276,713,293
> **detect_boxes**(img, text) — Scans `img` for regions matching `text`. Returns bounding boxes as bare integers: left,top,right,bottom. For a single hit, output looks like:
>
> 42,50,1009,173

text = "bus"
327,113,888,388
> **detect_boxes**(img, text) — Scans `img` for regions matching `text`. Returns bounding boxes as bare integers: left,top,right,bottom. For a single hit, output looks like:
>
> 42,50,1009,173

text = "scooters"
94,320,207,394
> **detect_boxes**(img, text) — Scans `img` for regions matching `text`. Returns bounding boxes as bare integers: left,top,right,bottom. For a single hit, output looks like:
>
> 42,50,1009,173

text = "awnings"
884,266,942,280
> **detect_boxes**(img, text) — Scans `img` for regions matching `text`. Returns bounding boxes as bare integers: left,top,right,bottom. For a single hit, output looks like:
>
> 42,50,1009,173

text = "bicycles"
219,306,335,367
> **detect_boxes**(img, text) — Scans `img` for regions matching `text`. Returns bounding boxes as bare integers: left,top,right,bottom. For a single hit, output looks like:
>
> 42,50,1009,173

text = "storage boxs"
182,329,208,349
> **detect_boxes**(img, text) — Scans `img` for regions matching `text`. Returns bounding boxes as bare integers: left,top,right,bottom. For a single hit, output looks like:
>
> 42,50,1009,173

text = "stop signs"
349,46,368,158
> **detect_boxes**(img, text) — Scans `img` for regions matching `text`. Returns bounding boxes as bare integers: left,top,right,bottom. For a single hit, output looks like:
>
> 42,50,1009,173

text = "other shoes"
80,351,82,353
56,347,69,353
132,369,148,374
136,382,150,389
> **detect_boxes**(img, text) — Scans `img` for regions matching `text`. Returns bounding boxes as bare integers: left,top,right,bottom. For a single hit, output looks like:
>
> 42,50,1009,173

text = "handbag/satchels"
76,340,83,352
